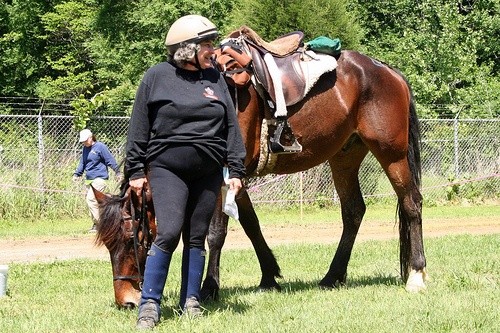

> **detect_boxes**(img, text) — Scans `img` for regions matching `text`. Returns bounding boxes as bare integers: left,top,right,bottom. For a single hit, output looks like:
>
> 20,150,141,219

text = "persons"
124,16,246,329
72,129,121,232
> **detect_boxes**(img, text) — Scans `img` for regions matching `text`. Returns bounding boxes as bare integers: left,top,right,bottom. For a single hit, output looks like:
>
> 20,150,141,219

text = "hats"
79,128,93,142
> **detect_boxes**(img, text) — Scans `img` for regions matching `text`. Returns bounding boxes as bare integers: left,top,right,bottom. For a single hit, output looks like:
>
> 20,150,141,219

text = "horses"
89,48,427,313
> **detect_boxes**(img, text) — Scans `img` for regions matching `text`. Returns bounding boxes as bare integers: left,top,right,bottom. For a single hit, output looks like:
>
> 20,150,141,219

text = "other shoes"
179,297,201,316
90,222,98,231
137,299,161,329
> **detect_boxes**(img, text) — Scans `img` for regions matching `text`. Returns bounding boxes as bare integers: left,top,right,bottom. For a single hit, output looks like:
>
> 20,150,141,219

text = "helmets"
165,14,219,50
